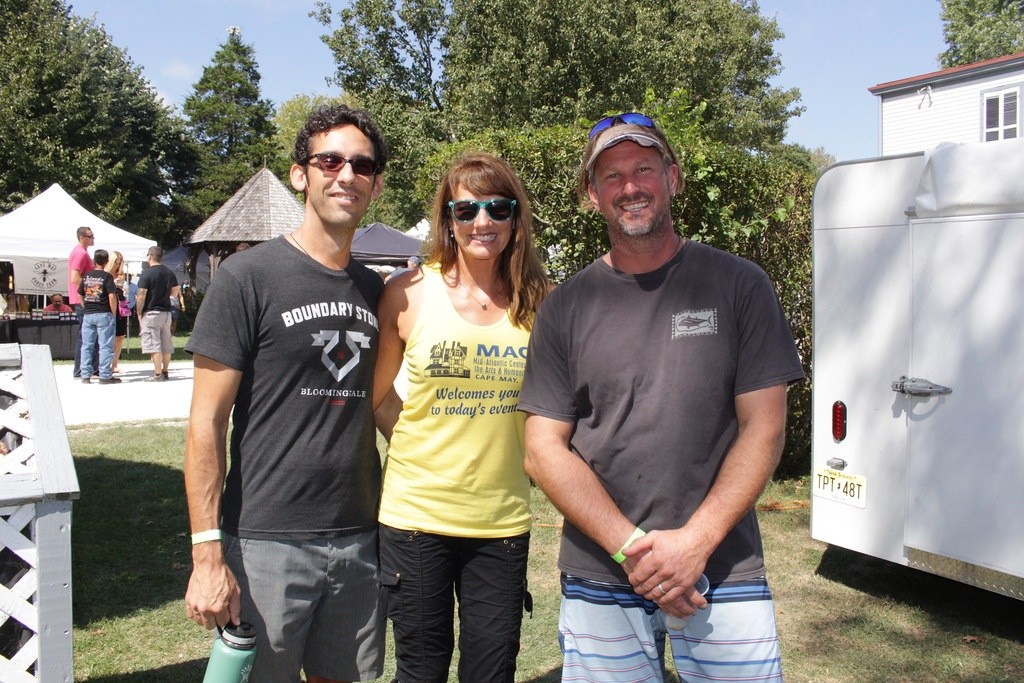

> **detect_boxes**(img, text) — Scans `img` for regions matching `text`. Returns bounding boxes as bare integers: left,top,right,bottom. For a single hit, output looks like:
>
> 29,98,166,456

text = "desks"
0,320,79,358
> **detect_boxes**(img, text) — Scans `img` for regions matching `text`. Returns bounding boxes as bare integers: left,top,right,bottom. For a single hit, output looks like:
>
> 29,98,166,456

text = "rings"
658,584,666,594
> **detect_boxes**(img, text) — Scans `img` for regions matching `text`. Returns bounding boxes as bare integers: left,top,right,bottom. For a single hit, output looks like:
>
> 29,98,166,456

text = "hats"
586,124,673,184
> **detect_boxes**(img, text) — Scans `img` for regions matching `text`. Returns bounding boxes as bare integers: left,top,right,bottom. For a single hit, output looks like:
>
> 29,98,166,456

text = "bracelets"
191,528,222,545
611,527,647,564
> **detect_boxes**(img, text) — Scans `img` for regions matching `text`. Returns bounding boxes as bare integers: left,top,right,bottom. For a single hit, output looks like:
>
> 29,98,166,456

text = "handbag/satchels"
118,300,132,317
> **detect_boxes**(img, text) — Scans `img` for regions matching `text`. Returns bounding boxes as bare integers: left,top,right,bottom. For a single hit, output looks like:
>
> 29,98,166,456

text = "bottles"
203,619,257,683
123,281,129,297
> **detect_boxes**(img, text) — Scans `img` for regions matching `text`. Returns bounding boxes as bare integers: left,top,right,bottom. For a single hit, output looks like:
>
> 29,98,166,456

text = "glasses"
448,197,518,223
588,112,679,167
84,234,94,239
298,153,381,176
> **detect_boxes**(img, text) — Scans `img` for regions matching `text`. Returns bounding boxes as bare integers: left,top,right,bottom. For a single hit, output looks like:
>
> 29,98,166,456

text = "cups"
663,573,710,633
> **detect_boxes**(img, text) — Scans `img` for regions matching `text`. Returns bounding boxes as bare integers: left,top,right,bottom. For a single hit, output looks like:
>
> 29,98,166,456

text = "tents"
350,222,431,267
0,183,158,355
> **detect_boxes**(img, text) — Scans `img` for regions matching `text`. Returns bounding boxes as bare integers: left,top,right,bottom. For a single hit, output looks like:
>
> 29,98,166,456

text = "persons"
68,226,100,377
43,294,73,313
195,241,253,293
183,103,388,683
76,249,122,384
369,154,562,683
385,255,420,282
104,246,185,383
9,294,30,313
516,112,807,682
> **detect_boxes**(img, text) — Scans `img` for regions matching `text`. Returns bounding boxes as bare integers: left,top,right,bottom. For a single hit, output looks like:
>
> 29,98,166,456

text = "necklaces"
459,283,505,310
666,236,681,261
290,232,311,256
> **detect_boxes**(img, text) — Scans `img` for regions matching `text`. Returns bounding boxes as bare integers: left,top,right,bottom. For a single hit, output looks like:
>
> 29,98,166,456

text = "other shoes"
162,370,168,380
111,368,121,373
81,378,90,384
144,373,164,382
99,377,122,383
92,370,99,376
73,373,81,380
